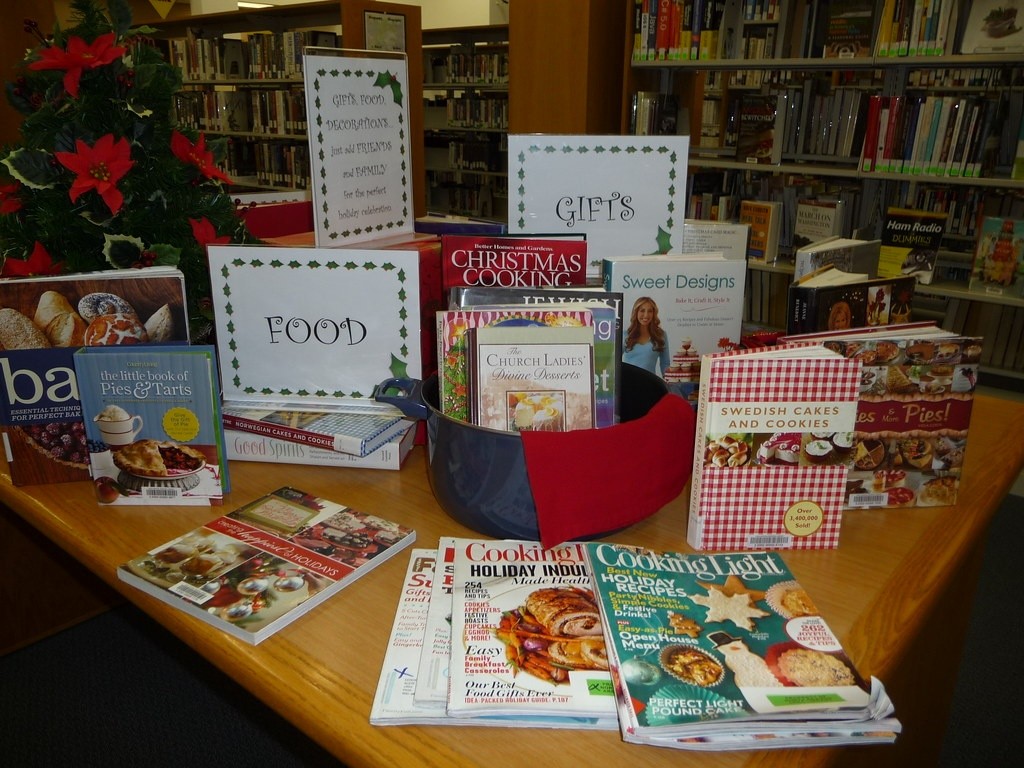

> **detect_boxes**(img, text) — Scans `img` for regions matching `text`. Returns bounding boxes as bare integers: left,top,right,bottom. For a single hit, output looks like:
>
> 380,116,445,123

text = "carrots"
500,615,560,680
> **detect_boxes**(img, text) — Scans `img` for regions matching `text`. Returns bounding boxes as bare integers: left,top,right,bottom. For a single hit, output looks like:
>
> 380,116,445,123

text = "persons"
622,297,671,375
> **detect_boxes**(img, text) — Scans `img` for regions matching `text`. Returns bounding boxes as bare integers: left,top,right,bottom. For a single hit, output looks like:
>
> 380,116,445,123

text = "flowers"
0,3,255,342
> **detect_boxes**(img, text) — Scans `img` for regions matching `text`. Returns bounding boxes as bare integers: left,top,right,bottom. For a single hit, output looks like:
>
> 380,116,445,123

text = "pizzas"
846,342,900,364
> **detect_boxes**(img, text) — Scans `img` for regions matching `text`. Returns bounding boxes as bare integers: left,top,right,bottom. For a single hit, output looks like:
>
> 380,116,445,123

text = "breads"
703,436,751,467
0,291,172,348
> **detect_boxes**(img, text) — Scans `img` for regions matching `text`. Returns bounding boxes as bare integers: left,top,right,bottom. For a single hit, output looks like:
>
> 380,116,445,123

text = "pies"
114,439,207,477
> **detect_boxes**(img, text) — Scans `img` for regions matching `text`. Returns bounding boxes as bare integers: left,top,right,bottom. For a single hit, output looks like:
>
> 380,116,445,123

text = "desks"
0,271,1024,768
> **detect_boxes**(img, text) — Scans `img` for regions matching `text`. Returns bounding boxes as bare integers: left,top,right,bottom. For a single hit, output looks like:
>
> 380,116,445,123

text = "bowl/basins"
860,371,877,392
824,341,845,354
804,432,853,463
873,470,907,488
964,348,982,356
879,487,917,508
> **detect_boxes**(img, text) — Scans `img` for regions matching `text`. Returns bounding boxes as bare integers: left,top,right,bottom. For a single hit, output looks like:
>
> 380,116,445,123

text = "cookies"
778,648,855,688
669,574,769,637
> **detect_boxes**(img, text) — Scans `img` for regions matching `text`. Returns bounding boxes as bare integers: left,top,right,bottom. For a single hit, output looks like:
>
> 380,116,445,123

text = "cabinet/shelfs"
116,2,420,192
511,1,1023,386
421,22,514,224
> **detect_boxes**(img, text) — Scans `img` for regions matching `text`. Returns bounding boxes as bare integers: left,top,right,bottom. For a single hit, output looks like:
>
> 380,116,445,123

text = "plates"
935,354,961,364
854,351,900,366
703,439,751,467
756,446,799,466
113,451,207,480
919,383,945,394
905,343,940,365
843,477,874,503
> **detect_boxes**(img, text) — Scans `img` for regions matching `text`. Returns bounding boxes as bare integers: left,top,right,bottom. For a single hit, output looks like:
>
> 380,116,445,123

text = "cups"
93,413,143,446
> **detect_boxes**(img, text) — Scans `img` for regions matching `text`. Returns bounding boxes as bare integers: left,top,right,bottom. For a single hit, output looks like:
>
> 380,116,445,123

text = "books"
368,536,902,752
631,0,1024,368
598,236,983,548
117,486,416,644
0,265,190,485
218,409,418,471
73,344,231,507
425,45,510,218
416,217,620,433
168,27,341,190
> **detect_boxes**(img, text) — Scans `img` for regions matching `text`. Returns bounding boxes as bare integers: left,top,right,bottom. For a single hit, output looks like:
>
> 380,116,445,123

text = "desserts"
906,343,981,376
766,580,820,619
659,644,725,687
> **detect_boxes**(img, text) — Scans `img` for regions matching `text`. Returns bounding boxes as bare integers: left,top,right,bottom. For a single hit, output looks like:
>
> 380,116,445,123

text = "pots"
376,362,672,541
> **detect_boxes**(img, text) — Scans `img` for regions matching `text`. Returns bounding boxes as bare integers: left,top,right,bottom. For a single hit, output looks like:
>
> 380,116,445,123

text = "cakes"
804,430,854,462
950,367,977,392
759,432,801,465
